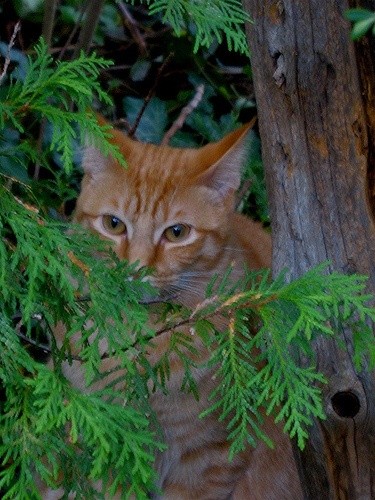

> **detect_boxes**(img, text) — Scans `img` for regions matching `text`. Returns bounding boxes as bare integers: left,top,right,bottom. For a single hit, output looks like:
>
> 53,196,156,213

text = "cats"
25,108,304,500
